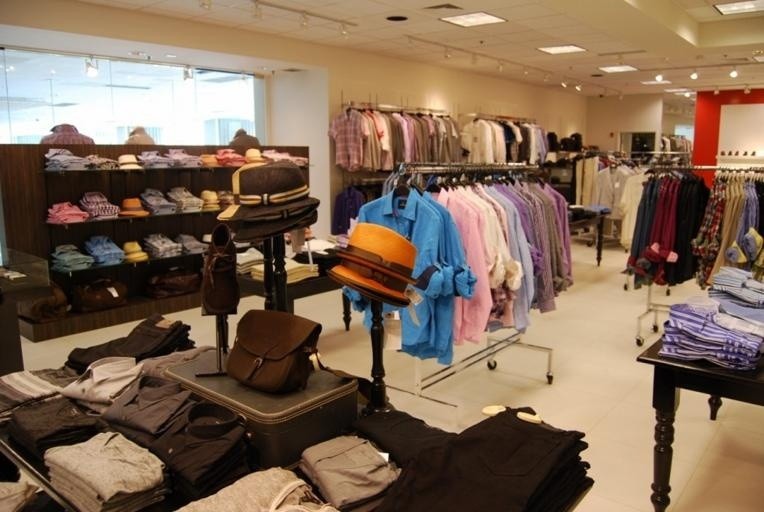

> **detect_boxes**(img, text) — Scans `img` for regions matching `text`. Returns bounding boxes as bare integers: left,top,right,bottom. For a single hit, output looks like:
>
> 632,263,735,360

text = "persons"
124,126,154,144
40,122,95,145
229,128,259,145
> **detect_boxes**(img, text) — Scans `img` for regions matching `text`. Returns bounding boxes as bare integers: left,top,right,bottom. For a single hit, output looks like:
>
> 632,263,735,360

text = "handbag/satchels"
69,277,129,311
224,309,321,393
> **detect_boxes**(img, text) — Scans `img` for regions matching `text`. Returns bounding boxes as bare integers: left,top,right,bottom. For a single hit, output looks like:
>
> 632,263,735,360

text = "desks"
0,263,56,481
636,335,764,512
220,253,351,349
568,213,611,266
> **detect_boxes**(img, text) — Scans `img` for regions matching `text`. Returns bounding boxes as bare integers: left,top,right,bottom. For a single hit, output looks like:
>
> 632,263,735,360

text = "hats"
119,198,150,217
117,154,143,171
215,160,321,242
201,189,220,212
324,223,417,308
122,241,149,263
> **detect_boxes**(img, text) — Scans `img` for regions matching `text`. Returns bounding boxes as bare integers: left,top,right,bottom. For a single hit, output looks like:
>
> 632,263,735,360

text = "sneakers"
202,224,241,315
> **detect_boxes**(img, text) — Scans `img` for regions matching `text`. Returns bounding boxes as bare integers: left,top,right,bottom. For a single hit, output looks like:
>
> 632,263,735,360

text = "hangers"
392,162,548,200
643,164,764,184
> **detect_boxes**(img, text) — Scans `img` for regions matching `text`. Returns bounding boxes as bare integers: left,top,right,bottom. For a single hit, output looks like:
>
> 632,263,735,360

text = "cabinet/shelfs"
0,142,310,344
